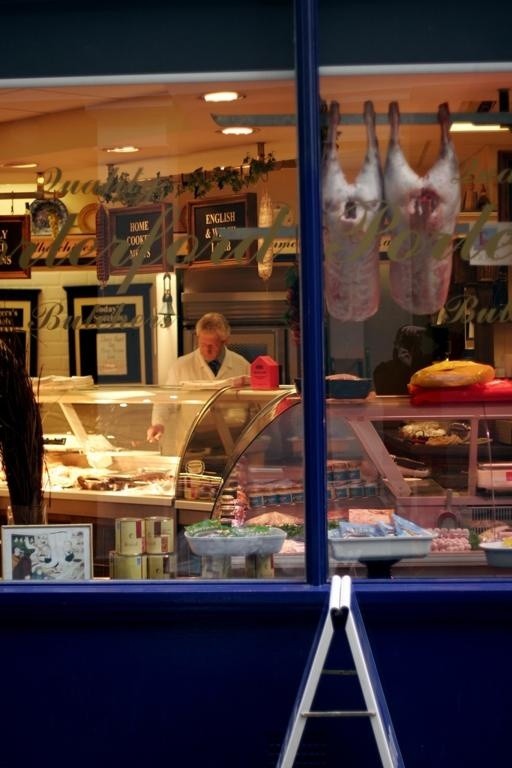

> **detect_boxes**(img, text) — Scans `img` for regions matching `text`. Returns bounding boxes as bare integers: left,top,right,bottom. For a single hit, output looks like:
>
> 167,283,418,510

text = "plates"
27,198,68,235
77,202,109,233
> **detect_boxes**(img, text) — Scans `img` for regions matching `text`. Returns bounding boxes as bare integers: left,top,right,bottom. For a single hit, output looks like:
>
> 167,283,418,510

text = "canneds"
115,517,145,557
247,484,305,508
145,554,178,580
326,461,381,499
146,518,175,554
109,550,147,580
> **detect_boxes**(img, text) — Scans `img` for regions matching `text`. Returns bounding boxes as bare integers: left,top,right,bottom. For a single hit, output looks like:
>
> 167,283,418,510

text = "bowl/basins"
292,375,374,399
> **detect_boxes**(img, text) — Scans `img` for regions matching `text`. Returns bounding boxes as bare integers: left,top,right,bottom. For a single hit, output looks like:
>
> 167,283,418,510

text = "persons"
145,312,252,474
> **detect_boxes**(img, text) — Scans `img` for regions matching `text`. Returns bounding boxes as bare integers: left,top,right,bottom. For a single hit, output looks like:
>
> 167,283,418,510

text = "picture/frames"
0,215,31,278
63,283,153,384
187,193,258,264
108,202,173,274
2,522,93,581
0,289,42,378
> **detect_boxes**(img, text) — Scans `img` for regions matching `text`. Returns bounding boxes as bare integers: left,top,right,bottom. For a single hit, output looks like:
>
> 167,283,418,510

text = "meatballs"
428,527,471,554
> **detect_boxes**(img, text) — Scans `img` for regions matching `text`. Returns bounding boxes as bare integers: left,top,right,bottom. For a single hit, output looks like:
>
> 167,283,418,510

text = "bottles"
478,184,492,211
